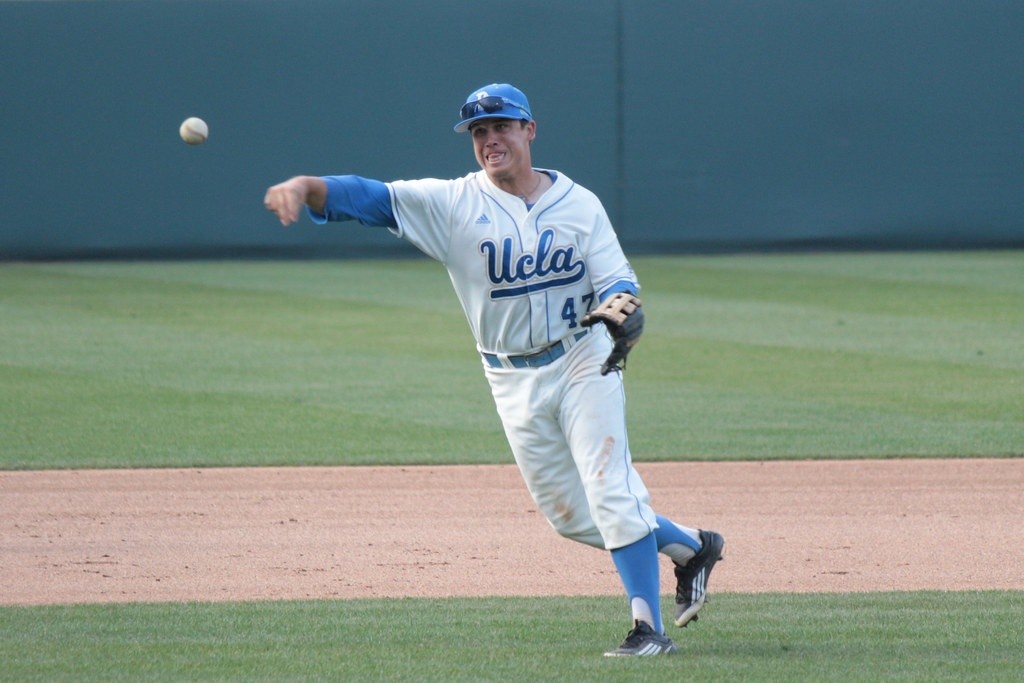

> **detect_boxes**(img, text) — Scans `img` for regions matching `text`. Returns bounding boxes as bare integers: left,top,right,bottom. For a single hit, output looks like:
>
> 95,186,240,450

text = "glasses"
461,96,529,122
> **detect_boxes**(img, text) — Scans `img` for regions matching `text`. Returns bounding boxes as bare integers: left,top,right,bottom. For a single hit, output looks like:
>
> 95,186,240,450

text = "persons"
264,81,726,657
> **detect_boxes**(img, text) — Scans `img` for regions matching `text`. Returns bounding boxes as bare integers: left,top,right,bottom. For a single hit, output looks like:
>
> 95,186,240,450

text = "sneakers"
604,618,681,659
673,528,726,628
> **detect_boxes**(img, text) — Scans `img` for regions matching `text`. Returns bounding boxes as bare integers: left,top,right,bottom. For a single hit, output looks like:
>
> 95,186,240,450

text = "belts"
482,328,588,369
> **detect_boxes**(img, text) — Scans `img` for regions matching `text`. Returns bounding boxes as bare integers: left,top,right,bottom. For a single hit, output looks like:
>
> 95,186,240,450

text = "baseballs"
179,117,209,145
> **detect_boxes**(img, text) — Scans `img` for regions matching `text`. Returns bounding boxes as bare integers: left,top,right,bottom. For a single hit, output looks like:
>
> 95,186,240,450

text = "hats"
453,83,533,133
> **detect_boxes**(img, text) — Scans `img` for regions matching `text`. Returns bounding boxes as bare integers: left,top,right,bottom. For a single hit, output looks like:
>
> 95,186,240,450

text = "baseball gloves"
580,292,645,376
